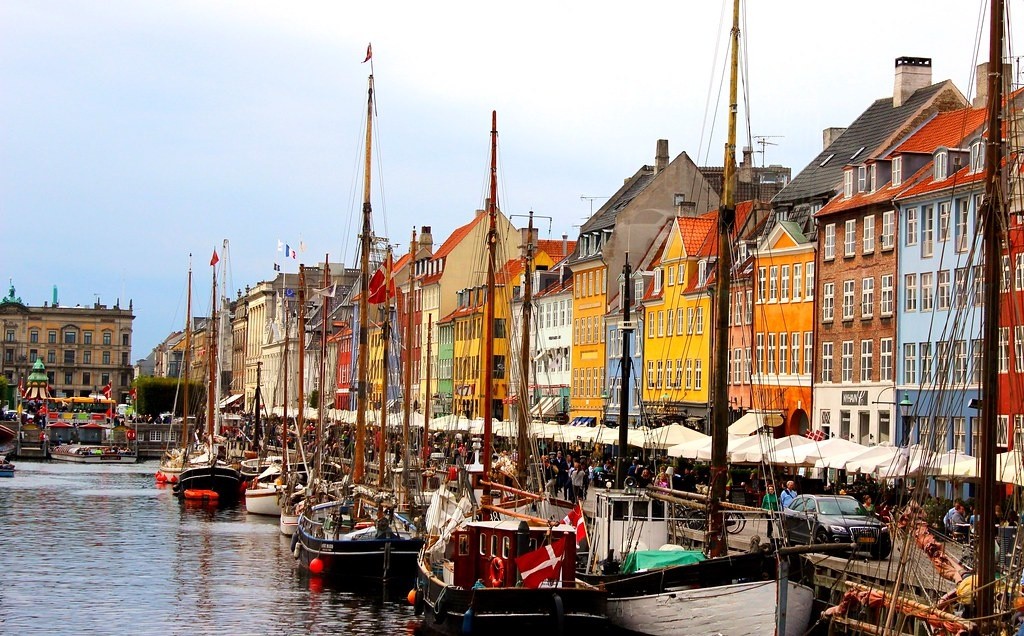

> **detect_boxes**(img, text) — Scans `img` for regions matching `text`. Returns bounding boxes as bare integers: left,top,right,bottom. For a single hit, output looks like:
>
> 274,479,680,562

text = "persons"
40,431,64,449
943,501,1020,544
222,408,879,539
114,415,171,427
3,453,11,464
0,398,111,424
80,446,131,460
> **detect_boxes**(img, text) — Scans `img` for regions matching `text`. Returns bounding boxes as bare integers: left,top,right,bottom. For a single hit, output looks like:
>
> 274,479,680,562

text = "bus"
42,397,117,424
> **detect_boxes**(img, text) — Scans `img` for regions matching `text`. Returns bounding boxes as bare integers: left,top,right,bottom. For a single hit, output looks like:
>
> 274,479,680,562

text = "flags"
129,387,137,400
286,244,296,259
560,504,586,543
103,382,111,400
274,262,280,271
210,251,219,265
514,537,565,589
368,254,395,304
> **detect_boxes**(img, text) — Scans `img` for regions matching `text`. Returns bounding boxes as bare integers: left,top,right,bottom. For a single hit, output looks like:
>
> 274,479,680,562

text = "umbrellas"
259,405,1024,511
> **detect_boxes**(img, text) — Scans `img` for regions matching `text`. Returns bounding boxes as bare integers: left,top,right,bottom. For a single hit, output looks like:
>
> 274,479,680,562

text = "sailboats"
155,0,1024,635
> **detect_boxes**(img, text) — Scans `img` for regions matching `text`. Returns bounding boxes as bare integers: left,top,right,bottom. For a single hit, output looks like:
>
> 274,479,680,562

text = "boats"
0,458,15,478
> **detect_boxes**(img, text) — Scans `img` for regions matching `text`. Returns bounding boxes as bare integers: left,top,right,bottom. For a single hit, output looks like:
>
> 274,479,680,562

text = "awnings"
221,394,243,409
727,410,784,435
527,397,563,418
569,417,595,427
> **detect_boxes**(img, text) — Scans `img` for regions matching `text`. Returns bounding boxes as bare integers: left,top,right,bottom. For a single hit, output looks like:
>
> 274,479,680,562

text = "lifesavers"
251,477,258,490
354,522,374,529
490,556,505,587
